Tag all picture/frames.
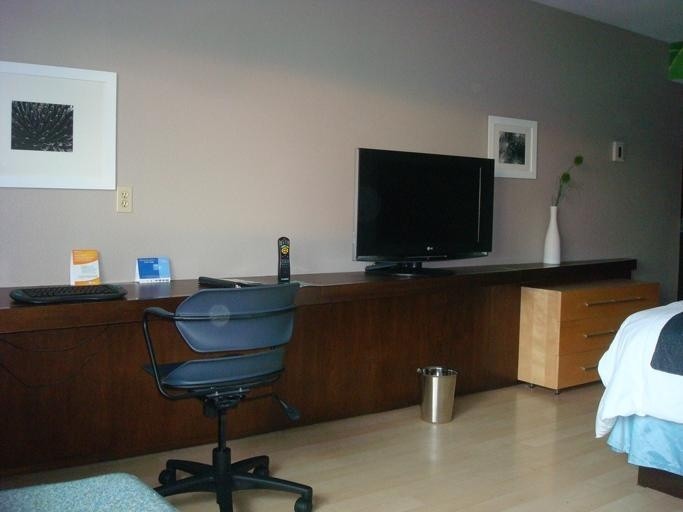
[0,60,119,193]
[486,114,538,181]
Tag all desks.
[0,257,638,480]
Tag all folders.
[200,276,260,289]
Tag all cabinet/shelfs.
[516,279,660,396]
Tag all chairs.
[139,282,312,511]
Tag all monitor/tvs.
[351,146,496,277]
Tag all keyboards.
[9,283,129,305]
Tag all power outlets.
[115,184,133,214]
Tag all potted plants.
[543,155,584,264]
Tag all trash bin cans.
[417,366,459,424]
